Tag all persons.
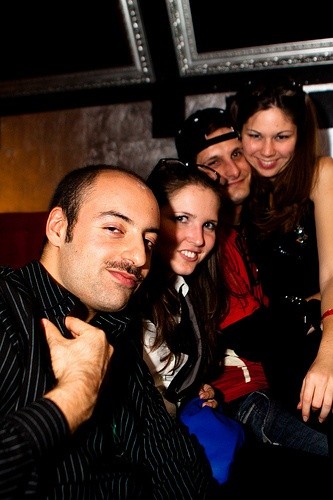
[142,156,245,481]
[168,75,333,500]
[0,162,234,500]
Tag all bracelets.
[320,308,333,318]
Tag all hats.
[176,108,241,163]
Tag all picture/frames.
[0,0,155,97]
[166,0,333,76]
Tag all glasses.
[148,159,222,188]
